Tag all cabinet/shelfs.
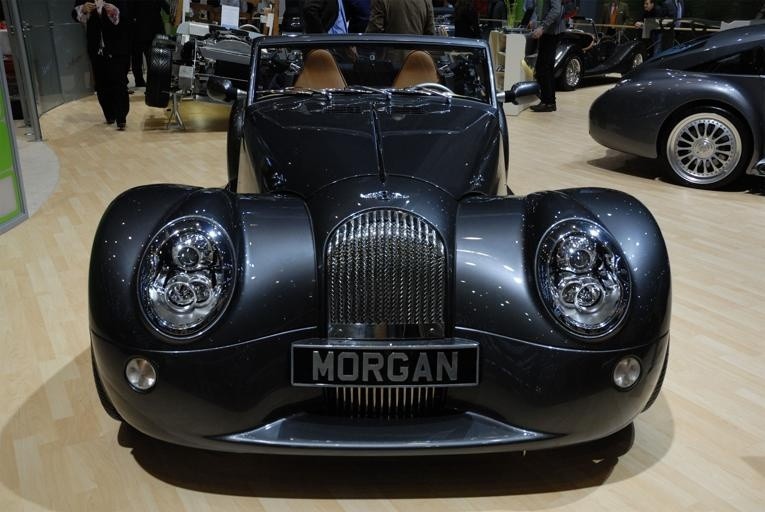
[488,29,540,116]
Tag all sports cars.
[589,24,765,188]
[554,18,645,90]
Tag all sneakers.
[106,118,127,128]
[530,99,557,113]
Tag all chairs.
[391,50,440,93]
[291,50,349,90]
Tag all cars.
[89,33,670,456]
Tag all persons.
[659,0,689,48]
[127,0,170,87]
[597,0,631,40]
[526,0,567,113]
[300,0,358,62]
[71,0,131,131]
[633,0,665,61]
[451,1,481,96]
[489,0,508,29]
[366,1,436,71]
